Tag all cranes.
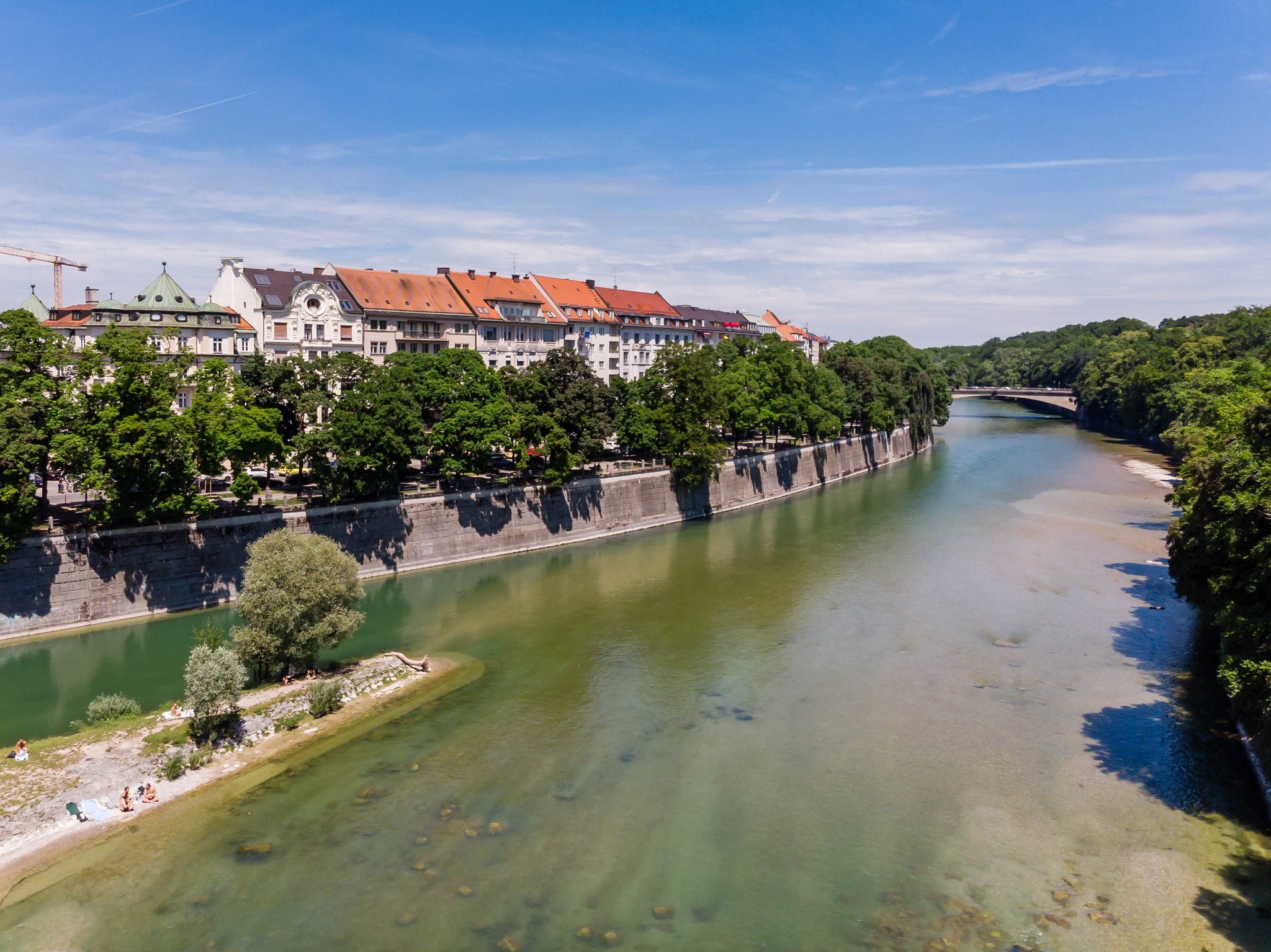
[0,245,89,309]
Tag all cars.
[192,479,232,494]
[188,476,198,483]
[605,441,620,451]
[241,466,276,478]
[970,386,978,389]
[420,446,550,474]
[28,473,43,487]
[46,469,62,481]
[326,461,339,470]
[285,473,320,486]
[1001,387,1011,389]
[387,464,420,475]
[198,474,232,481]
[601,446,630,457]
[278,465,309,477]
[251,476,284,487]
[714,423,763,441]
[1045,387,1052,391]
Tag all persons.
[59,478,74,493]
[15,739,28,757]
[283,674,295,685]
[142,783,159,804]
[591,463,602,473]
[120,786,135,812]
[171,703,181,717]
[751,439,756,453]
[306,668,316,680]
